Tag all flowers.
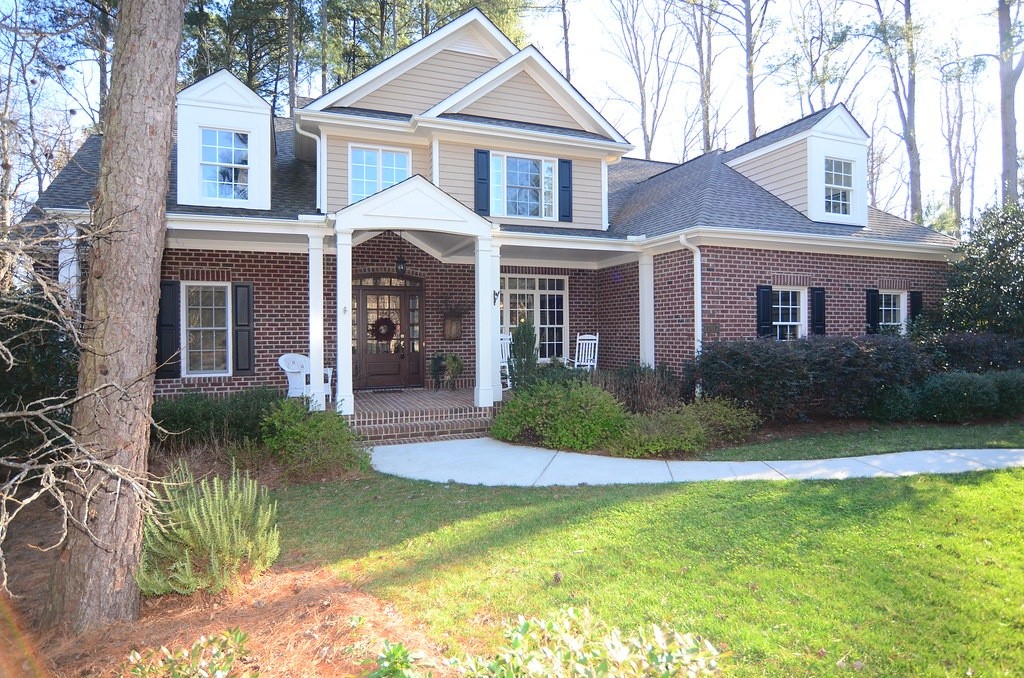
[369,317,396,342]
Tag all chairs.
[563,333,599,370]
[497,332,512,391]
[278,352,333,406]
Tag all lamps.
[395,228,408,274]
